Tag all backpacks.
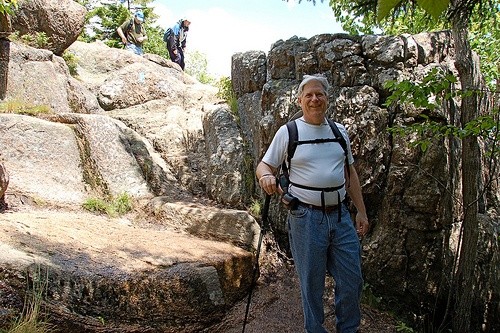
[163,27,173,42]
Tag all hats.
[134,11,145,22]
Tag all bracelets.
[261,174,272,177]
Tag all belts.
[300,200,349,211]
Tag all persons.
[255,75,369,333]
[117,12,148,56]
[167,16,192,71]
